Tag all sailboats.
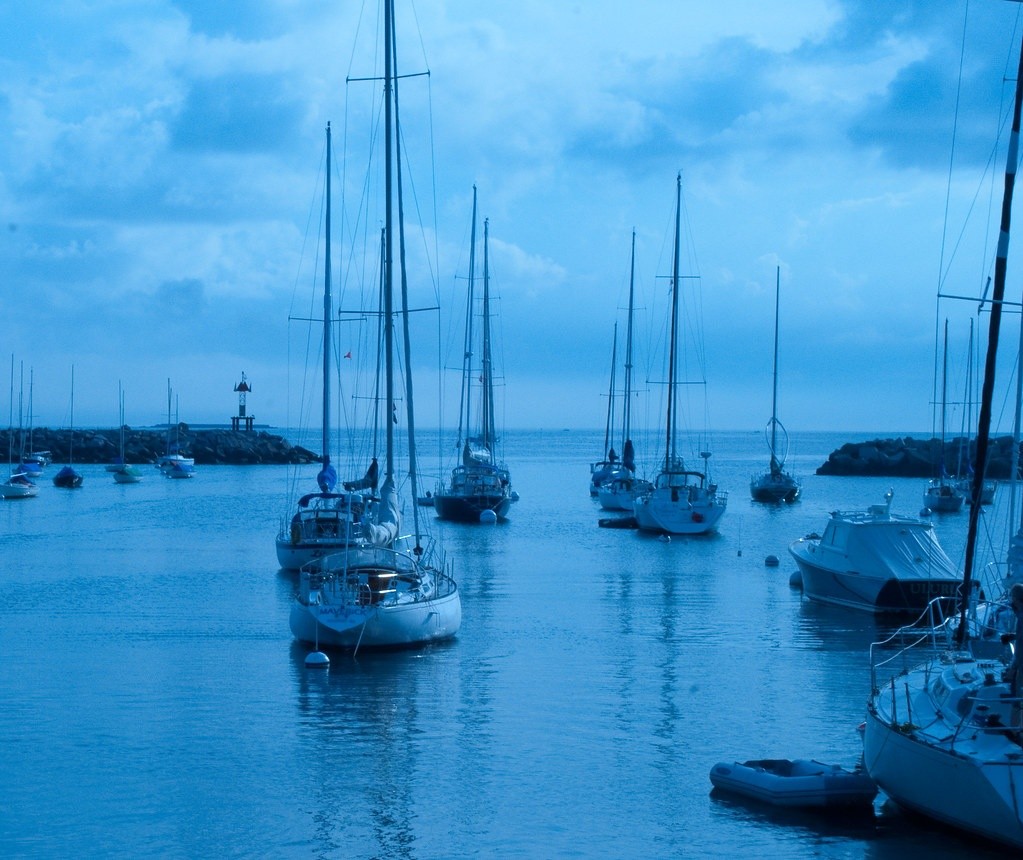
[750,265,803,503]
[160,394,197,479]
[154,377,195,469]
[113,390,145,484]
[290,0,460,649]
[924,318,966,512]
[435,218,513,523]
[856,0,1023,860]
[599,228,655,511]
[52,365,84,488]
[0,354,41,498]
[590,321,636,497]
[276,121,404,574]
[11,365,52,476]
[632,170,728,536]
[416,183,477,507]
[105,380,132,471]
[953,318,995,504]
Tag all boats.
[710,759,878,807]
[789,486,986,616]
